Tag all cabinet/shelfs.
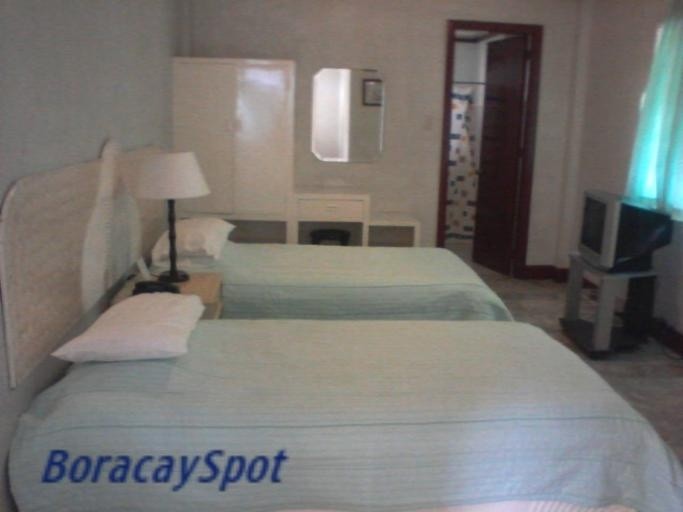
[172,56,295,244]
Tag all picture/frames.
[363,79,382,106]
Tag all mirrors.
[311,68,385,161]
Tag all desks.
[293,185,420,247]
[560,254,659,360]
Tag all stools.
[310,230,349,246]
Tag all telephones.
[132,281,180,297]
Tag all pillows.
[152,216,237,262]
[51,292,205,364]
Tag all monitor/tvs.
[579,190,671,272]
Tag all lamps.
[132,153,210,282]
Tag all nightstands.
[111,274,223,320]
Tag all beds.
[7,319,683,512]
[147,243,514,320]
